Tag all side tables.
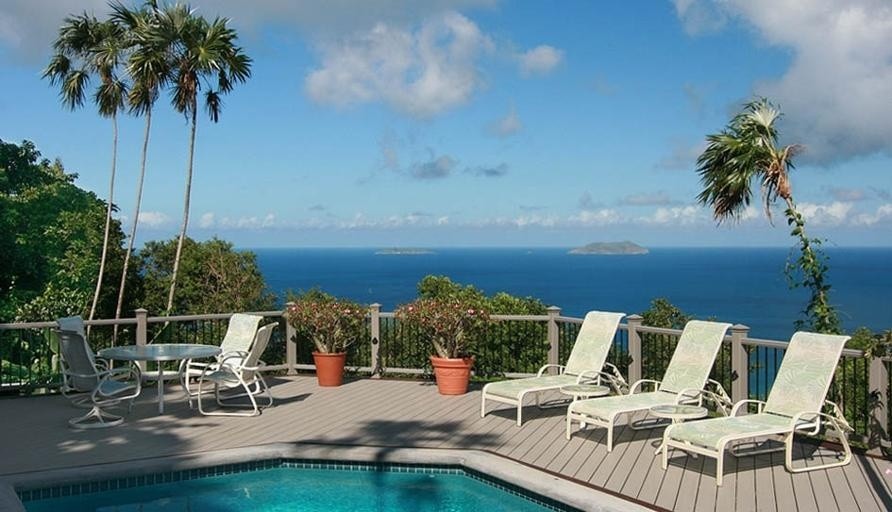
[560,384,610,429]
[648,404,708,459]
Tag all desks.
[97,342,222,414]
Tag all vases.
[430,355,476,396]
[312,352,347,387]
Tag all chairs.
[567,319,734,452]
[185,313,279,418]
[481,310,627,427]
[51,315,138,429]
[663,331,854,485]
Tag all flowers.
[393,295,484,358]
[282,297,370,354]
